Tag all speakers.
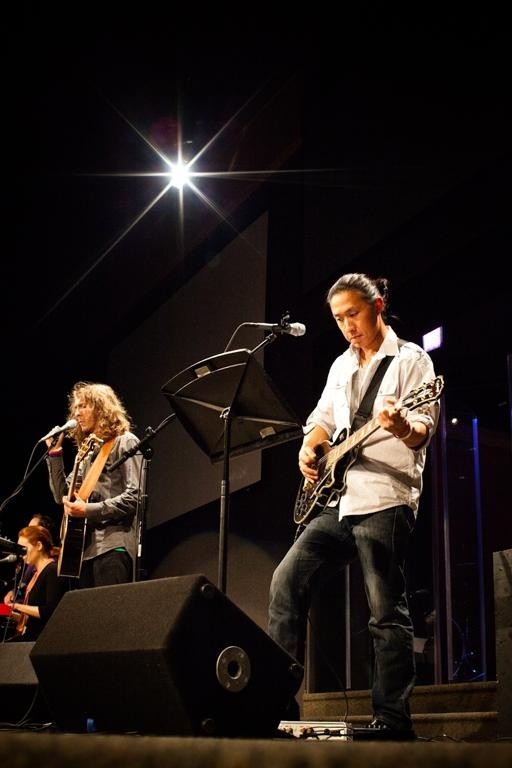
[27,572,307,734]
[0,639,52,729]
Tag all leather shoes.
[369,719,416,742]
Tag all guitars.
[56,431,105,579]
[0,602,28,635]
[293,375,446,525]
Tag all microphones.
[245,317,310,339]
[0,546,18,571]
[147,409,180,437]
[39,419,79,442]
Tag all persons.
[1,525,78,648]
[28,513,61,555]
[40,378,153,588]
[258,269,444,744]
